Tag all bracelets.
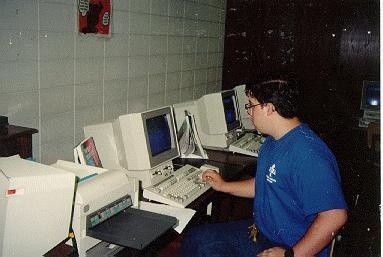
[284,248,294,257]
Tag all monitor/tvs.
[172,90,241,135]
[84,105,180,171]
[360,80,380,111]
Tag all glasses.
[245,104,261,117]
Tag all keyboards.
[229,133,266,157]
[143,165,213,208]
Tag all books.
[73,136,103,168]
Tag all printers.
[48,162,177,257]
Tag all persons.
[180,73,348,257]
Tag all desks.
[114,150,257,257]
